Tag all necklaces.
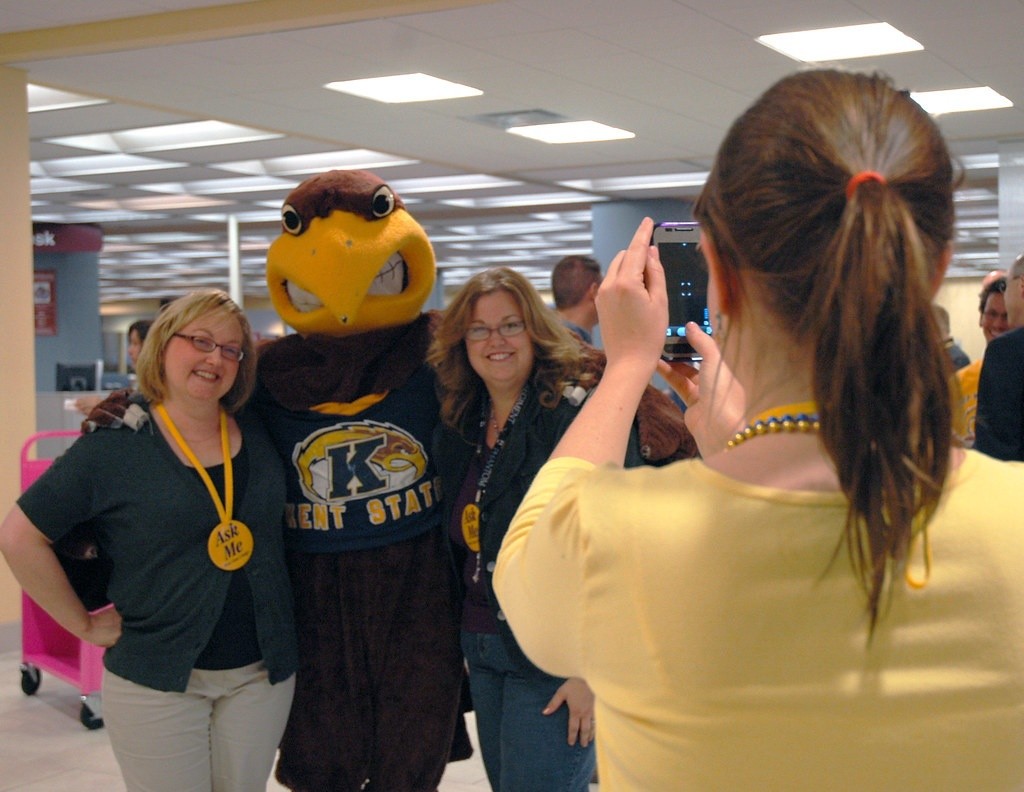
[724,402,821,451]
[184,427,218,443]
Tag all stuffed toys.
[81,169,697,792]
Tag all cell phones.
[650,222,713,363]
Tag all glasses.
[464,321,525,341]
[172,333,244,362]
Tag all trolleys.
[19,429,116,729]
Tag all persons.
[491,70,1024,792]
[0,287,300,792]
[550,256,603,345]
[424,268,646,792]
[127,319,151,365]
[930,256,1024,462]
